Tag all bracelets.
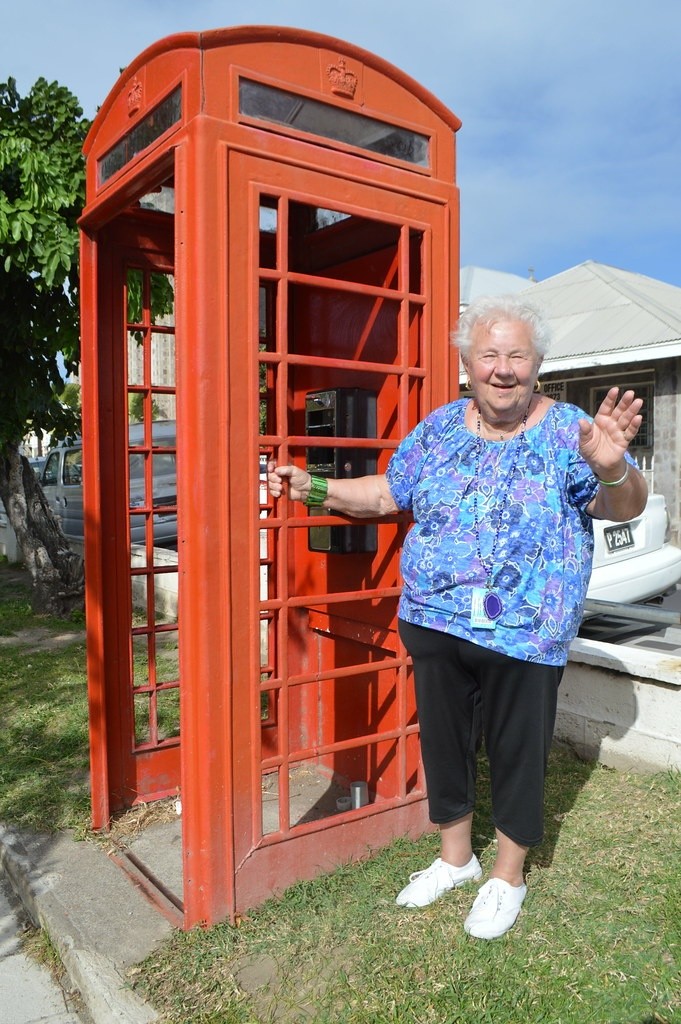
[303,475,328,508]
[593,463,630,487]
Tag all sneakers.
[396,853,482,908]
[464,878,528,940]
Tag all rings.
[624,433,631,441]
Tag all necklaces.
[483,417,520,440]
[475,402,530,617]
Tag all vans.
[36,419,177,545]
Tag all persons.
[258,293,648,941]
